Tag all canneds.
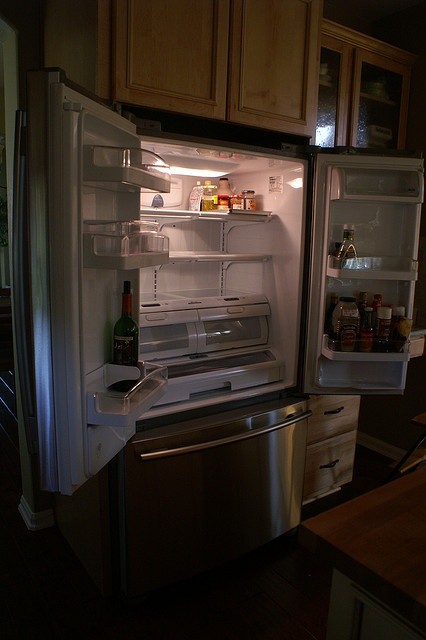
[217,194,229,210]
[360,326,374,351]
[242,190,255,211]
[229,194,242,210]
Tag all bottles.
[217,178,234,213]
[113,281,139,391]
[330,292,406,352]
[202,180,216,212]
[337,224,358,269]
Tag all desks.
[301,468,425,639]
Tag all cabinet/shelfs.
[312,19,412,147]
[44,0,321,138]
[304,393,360,505]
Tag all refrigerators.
[14,66,424,594]
